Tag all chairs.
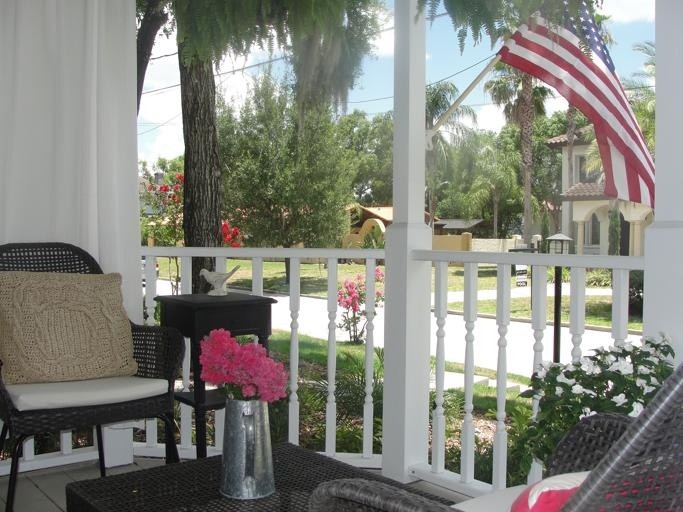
[0,243,185,512]
[309,362,682,512]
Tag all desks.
[66,442,460,512]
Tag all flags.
[493,3,654,209]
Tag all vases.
[218,398,276,500]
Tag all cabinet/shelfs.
[157,283,274,465]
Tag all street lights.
[544,234,573,363]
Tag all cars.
[141,257,159,286]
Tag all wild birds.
[199,265,240,289]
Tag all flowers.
[197,325,287,405]
